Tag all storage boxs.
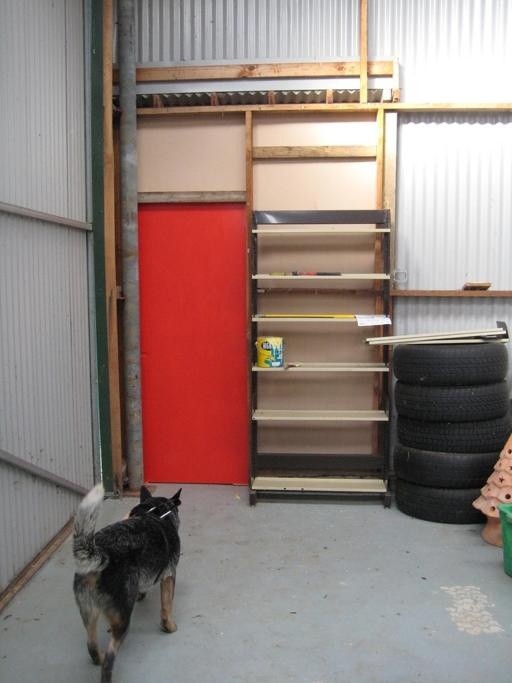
[496,502,512,578]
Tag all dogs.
[70,482,182,683]
